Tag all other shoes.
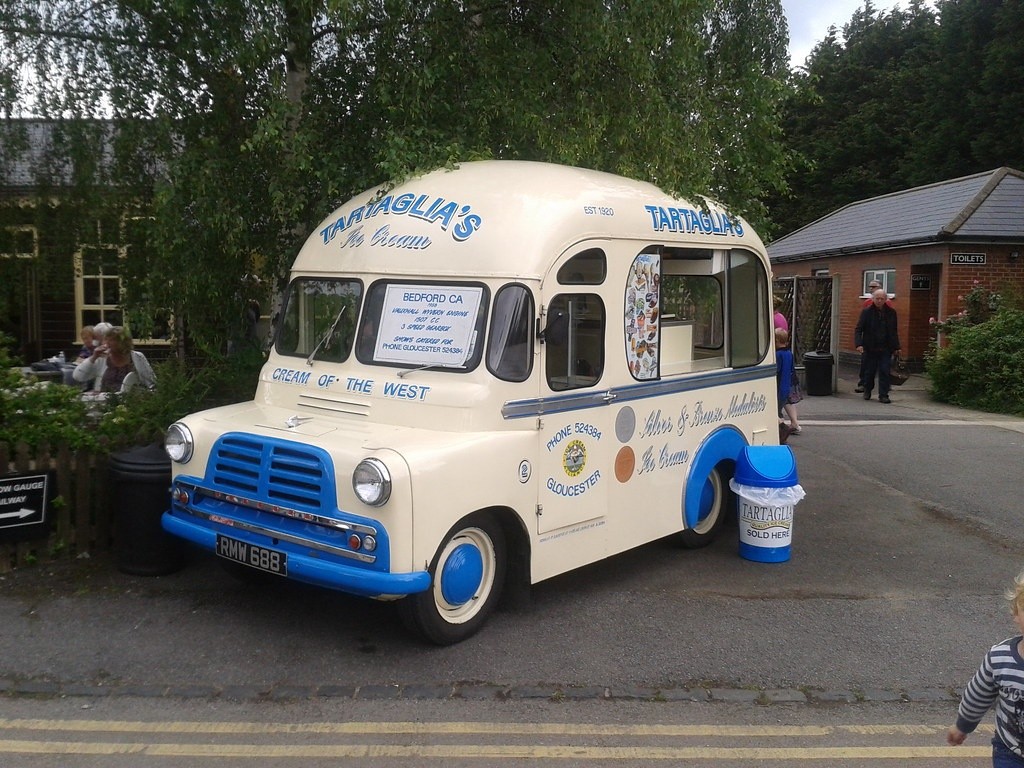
[790,426,802,435]
[855,385,866,393]
[863,388,872,400]
[878,393,891,404]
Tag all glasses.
[869,286,880,288]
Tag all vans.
[160,159,781,648]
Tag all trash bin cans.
[109,442,185,576]
[802,350,834,396]
[734,445,798,563]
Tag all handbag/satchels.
[887,353,910,386]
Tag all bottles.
[59,351,65,363]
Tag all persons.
[947,574,1024,768]
[854,289,900,403]
[774,310,803,434]
[72,323,158,392]
[774,328,793,422]
[855,279,894,393]
